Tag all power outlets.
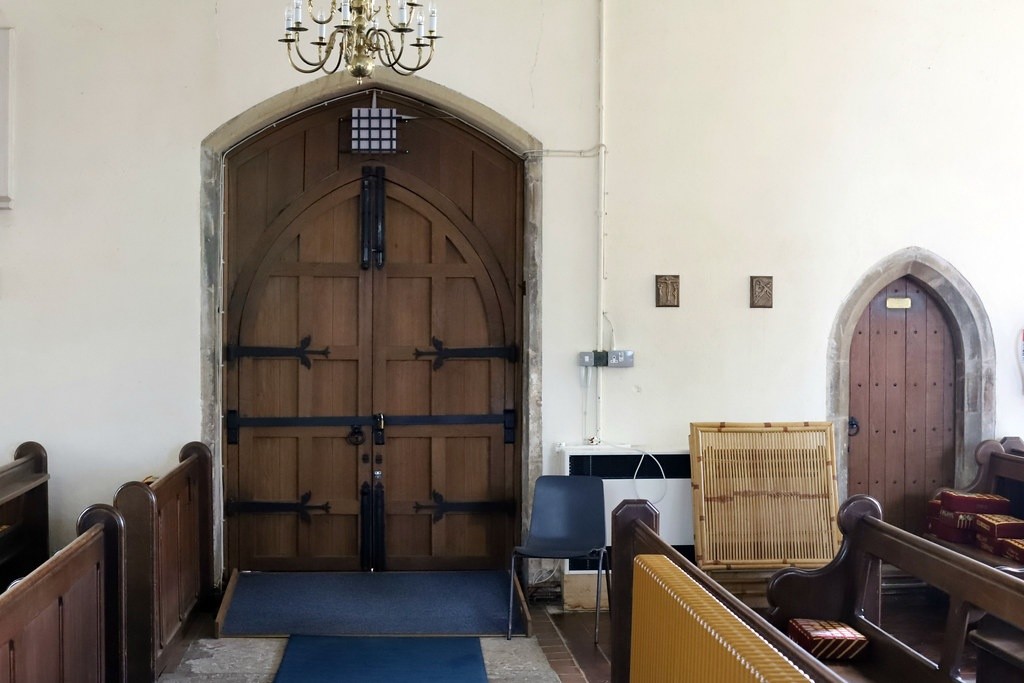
[607,351,636,366]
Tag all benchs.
[767,437,1024,683]
[0,441,212,683]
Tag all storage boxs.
[784,617,873,661]
[929,491,1024,563]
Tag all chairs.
[507,474,613,647]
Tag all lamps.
[280,1,442,88]
